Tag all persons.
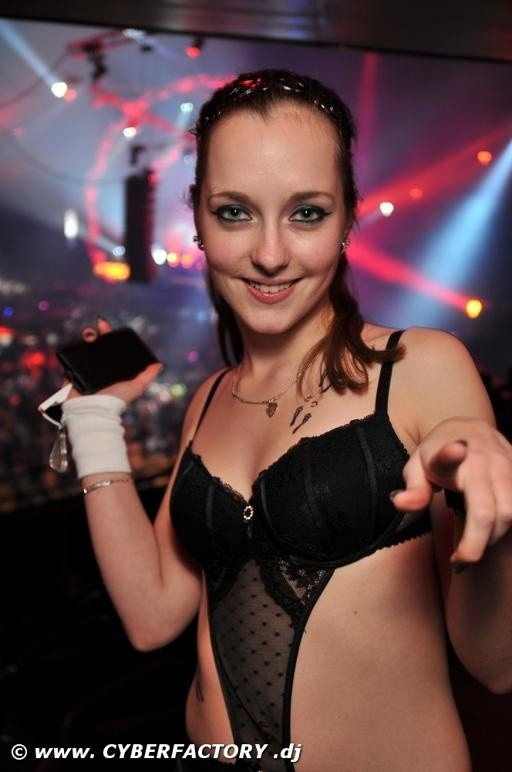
[48,67,512,772]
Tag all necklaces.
[231,358,309,417]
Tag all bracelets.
[80,478,135,494]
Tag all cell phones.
[54,323,155,391]
